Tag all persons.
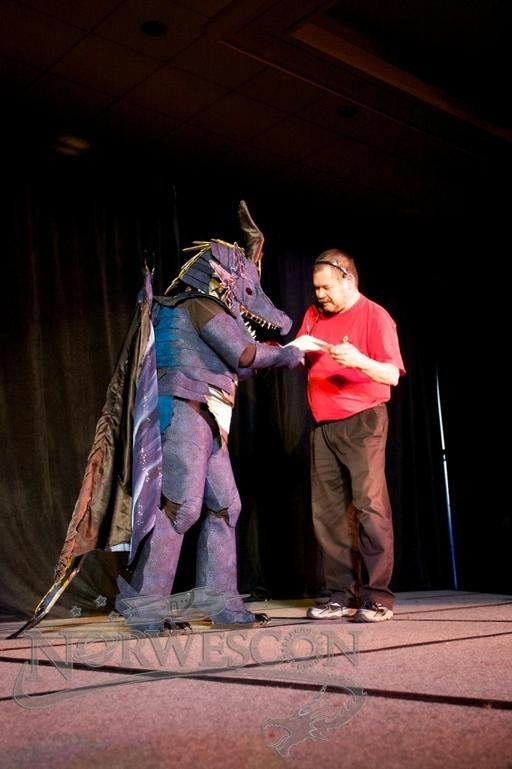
[7,199,306,637]
[289,248,401,623]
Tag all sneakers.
[307,600,358,619]
[355,600,394,623]
